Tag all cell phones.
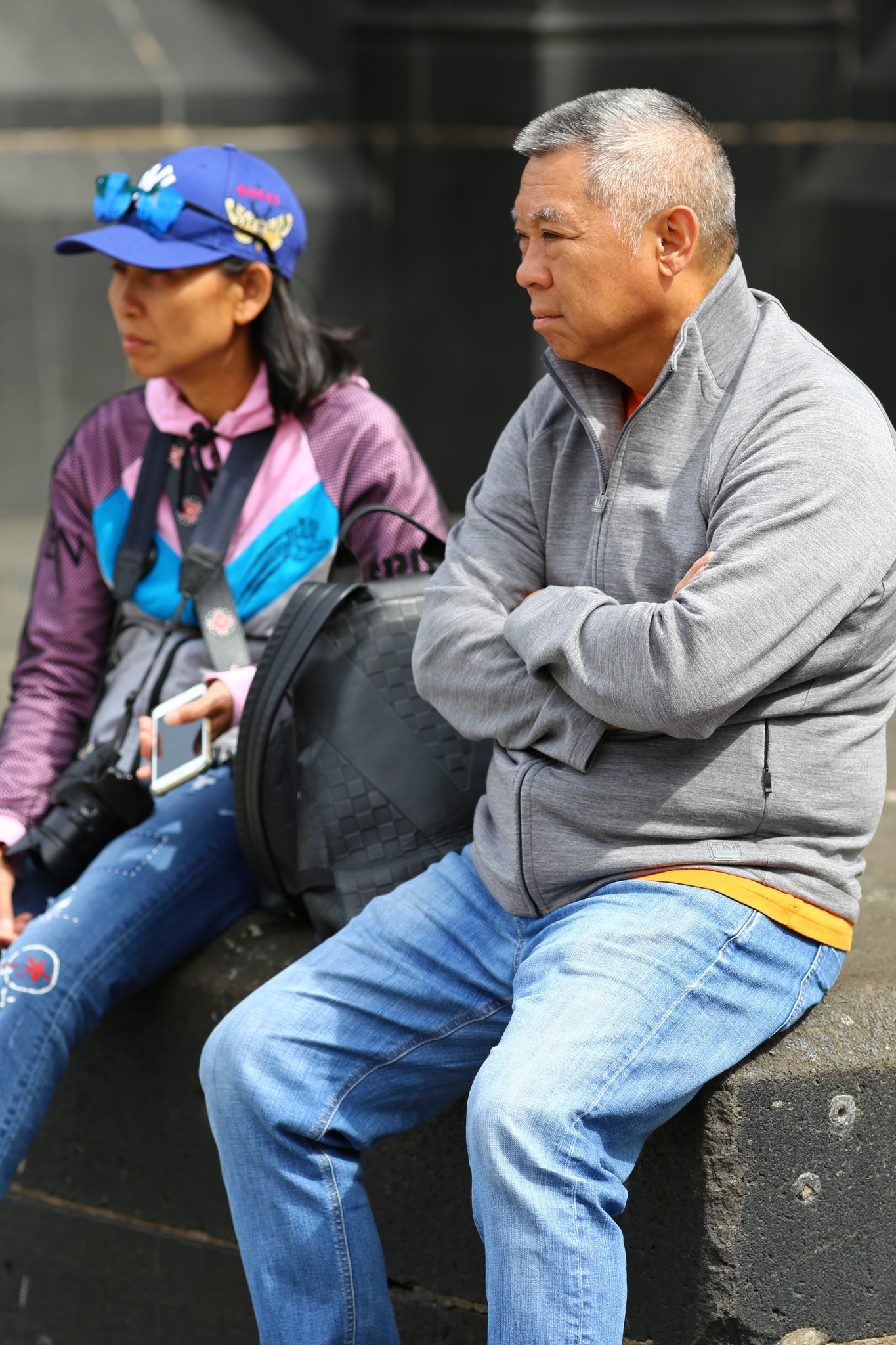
[150,682,214,797]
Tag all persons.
[197,86,896,1345]
[0,147,448,1179]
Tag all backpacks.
[233,503,496,944]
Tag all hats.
[54,143,308,282]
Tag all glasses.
[94,171,276,273]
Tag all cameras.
[2,769,157,898]
